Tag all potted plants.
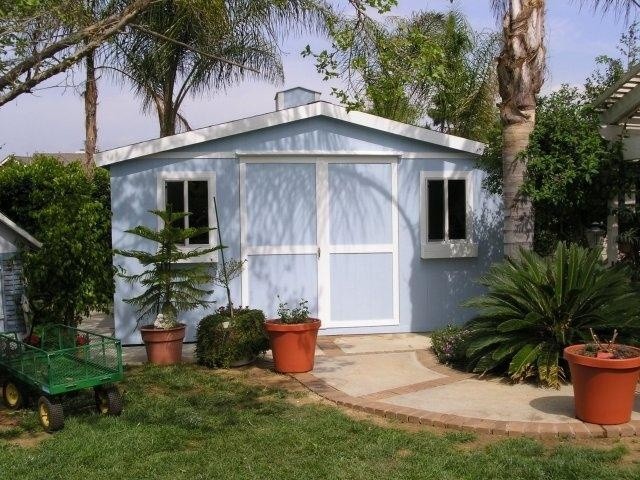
[564,326,640,424]
[113,202,322,378]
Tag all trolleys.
[1,323,125,434]
